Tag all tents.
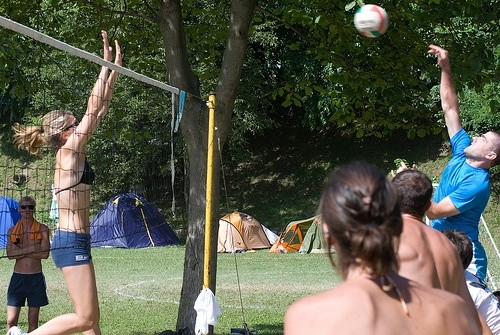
[89,192,180,249]
[0,195,22,248]
[217,211,284,253]
[268,213,336,254]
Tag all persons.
[442,229,500,335]
[391,169,483,335]
[7,197,51,333]
[5,32,123,335]
[392,44,500,283]
[284,160,478,334]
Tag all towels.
[194,287,221,335]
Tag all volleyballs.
[353,4,390,39]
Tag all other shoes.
[7,326,26,335]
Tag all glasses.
[63,121,79,131]
[21,205,35,209]
